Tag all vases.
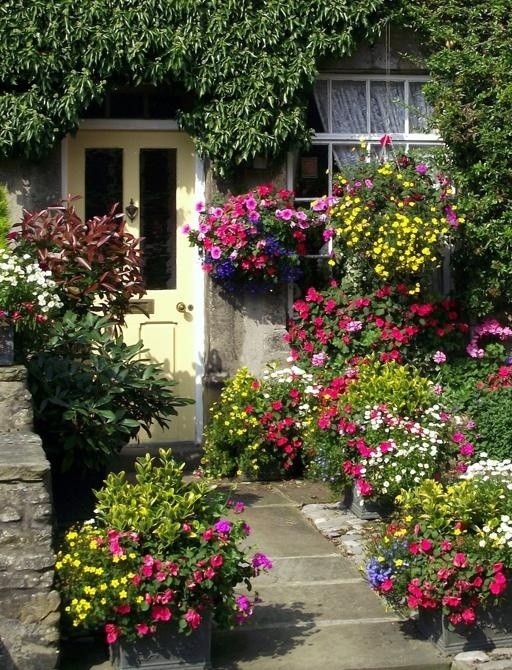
[416,602,512,657]
[108,614,213,670]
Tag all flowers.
[52,128,512,644]
